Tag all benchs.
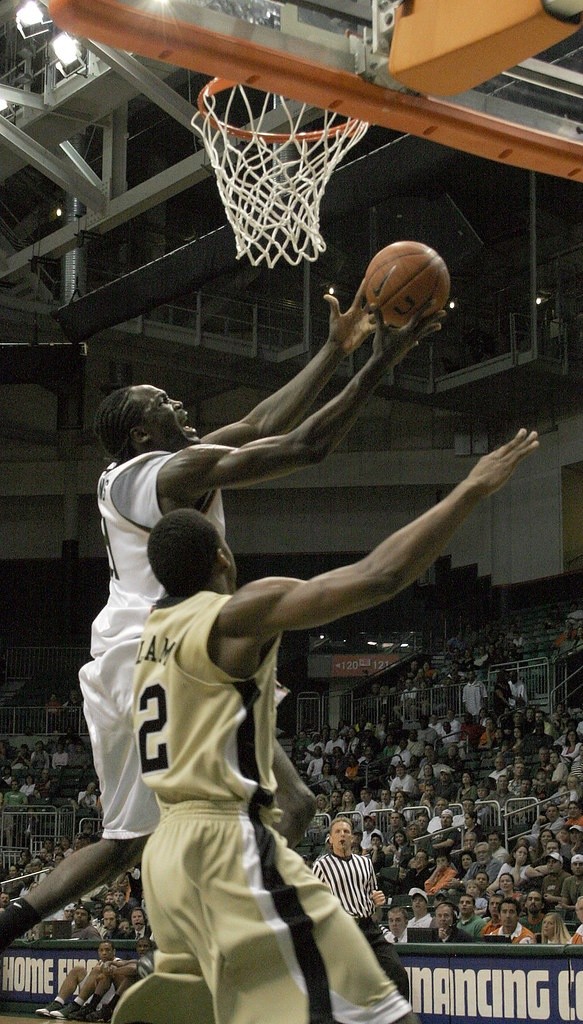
[0,595,583,933]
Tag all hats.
[434,888,449,899]
[440,767,451,776]
[545,852,563,864]
[537,767,547,773]
[77,904,92,921]
[409,888,428,903]
[64,903,76,911]
[364,816,376,825]
[571,854,583,864]
[568,825,583,832]
[442,809,453,818]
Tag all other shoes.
[86,1003,114,1023]
[69,1004,96,1020]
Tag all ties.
[394,937,398,943]
[135,933,140,940]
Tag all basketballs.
[363,240,451,327]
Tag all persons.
[132,429,537,1024]
[0,611,582,1021]
[2,278,442,942]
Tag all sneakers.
[50,1002,80,1019]
[35,1000,64,1016]
